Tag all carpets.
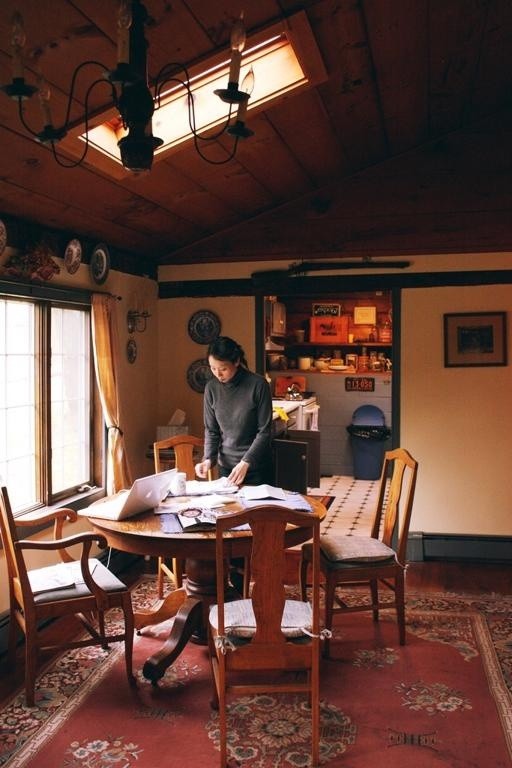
[306,494,337,513]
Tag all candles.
[133,301,148,314]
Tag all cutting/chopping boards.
[274,376,306,397]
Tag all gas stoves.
[270,395,316,406]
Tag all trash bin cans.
[346,405,386,480]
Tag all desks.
[83,491,329,693]
[144,442,199,472]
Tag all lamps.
[56,10,333,185]
[0,1,258,176]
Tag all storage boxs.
[310,315,349,344]
[311,303,340,317]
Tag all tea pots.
[285,383,304,401]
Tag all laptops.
[77,468,178,521]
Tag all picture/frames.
[353,306,376,324]
[442,308,508,370]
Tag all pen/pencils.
[162,490,172,503]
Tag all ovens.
[299,402,320,431]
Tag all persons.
[194,336,273,599]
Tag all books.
[175,507,217,532]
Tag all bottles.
[383,319,391,329]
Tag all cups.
[373,361,381,372]
[173,472,187,496]
[298,358,311,370]
[368,333,375,341]
[294,329,305,343]
[348,334,354,343]
[280,356,287,370]
[314,359,325,370]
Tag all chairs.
[0,484,139,707]
[207,503,322,768]
[297,447,420,660]
[151,433,216,600]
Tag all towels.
[310,404,319,431]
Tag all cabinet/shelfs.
[264,298,287,337]
[270,406,321,496]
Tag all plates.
[87,243,110,286]
[188,309,222,345]
[63,238,82,276]
[0,220,7,256]
[186,359,216,394]
[329,365,349,370]
[125,340,139,365]
[126,310,136,334]
